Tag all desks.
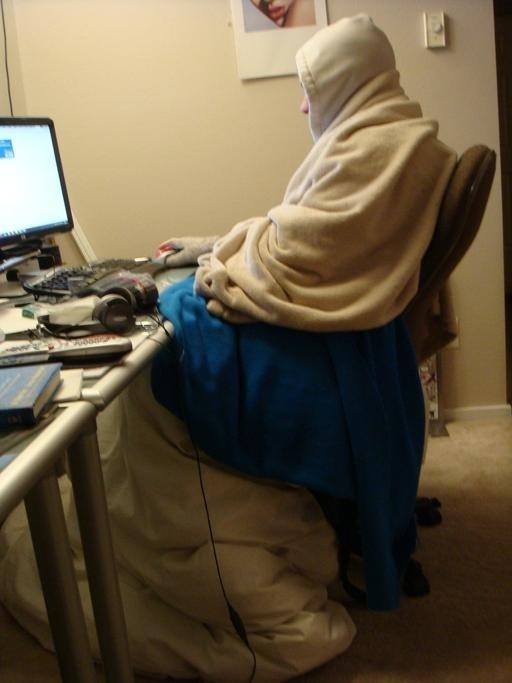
[1,262,203,683]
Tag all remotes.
[48,336,132,360]
[0,334,49,367]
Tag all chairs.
[154,138,498,594]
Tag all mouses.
[150,246,179,267]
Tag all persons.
[0,10,457,680]
[244,2,315,30]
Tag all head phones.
[37,286,138,338]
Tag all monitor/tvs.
[0,117,74,258]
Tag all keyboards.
[23,257,165,297]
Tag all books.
[0,356,85,457]
